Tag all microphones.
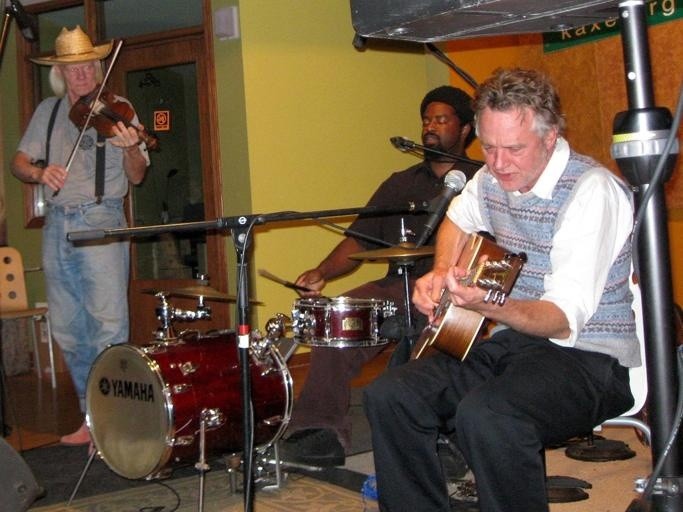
[414,169,467,250]
[390,135,416,152]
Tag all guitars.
[408,234,526,361]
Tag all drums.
[292,296,392,347]
[85,326,296,480]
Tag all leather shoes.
[60,421,91,444]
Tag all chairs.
[564,262,655,462]
[0,245,57,391]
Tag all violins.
[68,81,157,150]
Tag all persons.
[11,23,150,461]
[262,82,489,467]
[360,63,653,511]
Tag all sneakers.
[240,428,346,473]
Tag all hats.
[29,24,114,65]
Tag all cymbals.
[141,280,267,309]
[344,235,438,267]
